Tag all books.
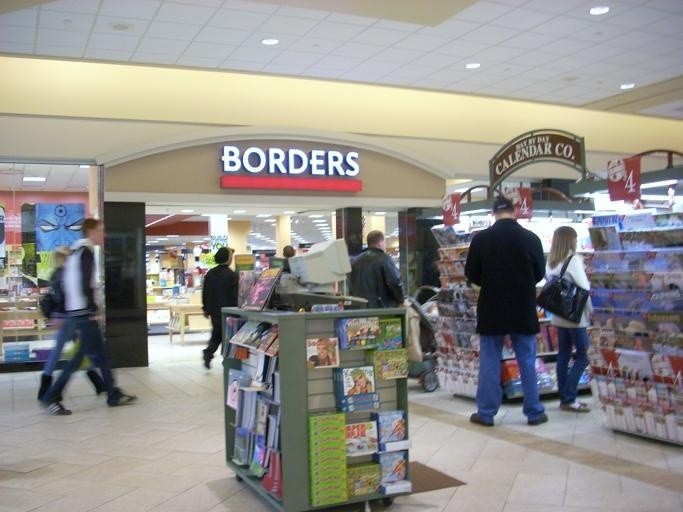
[220,314,281,501]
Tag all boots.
[86,370,107,395]
[37,373,65,402]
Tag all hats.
[214,248,235,265]
[493,197,513,212]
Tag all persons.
[347,368,372,396]
[40,218,137,416]
[280,246,297,275]
[199,246,238,370]
[347,230,405,308]
[462,198,548,427]
[307,338,337,367]
[36,245,122,402]
[542,225,594,414]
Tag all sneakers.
[562,400,591,413]
[559,400,587,409]
[106,388,139,406]
[39,398,71,414]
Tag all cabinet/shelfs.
[431,211,589,406]
[584,212,683,448]
[221,305,411,512]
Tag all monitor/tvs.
[288,238,352,293]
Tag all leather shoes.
[471,411,494,426]
[527,414,549,425]
[203,350,211,368]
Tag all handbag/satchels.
[537,275,590,323]
[41,292,53,316]
